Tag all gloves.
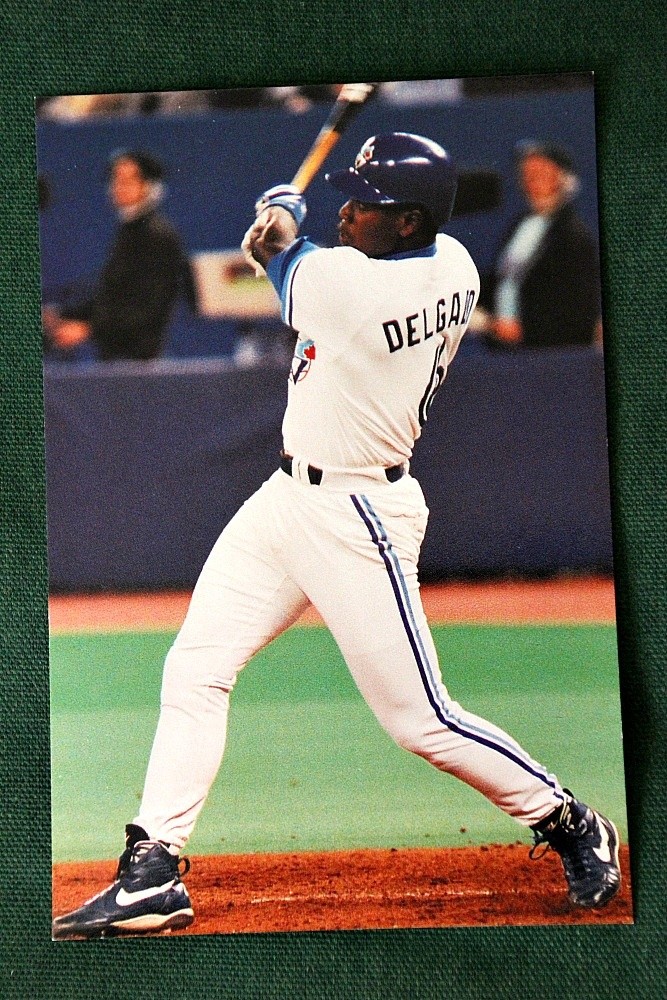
[253,183,307,226]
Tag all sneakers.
[529,788,621,908]
[51,824,195,938]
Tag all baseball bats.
[251,81,379,264]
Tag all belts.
[280,449,405,485]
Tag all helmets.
[324,133,457,225]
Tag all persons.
[467,136,600,350]
[50,127,623,941]
[42,148,185,363]
